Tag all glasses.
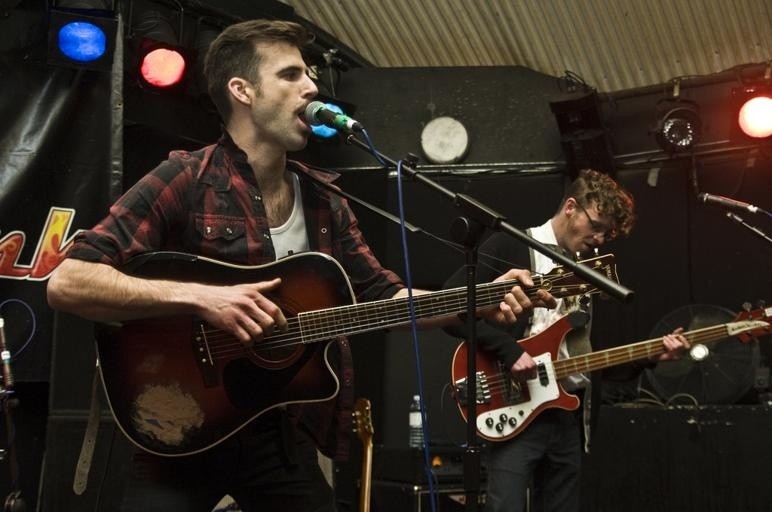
[575,197,615,243]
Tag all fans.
[644,302,761,411]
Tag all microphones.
[303,100,365,136]
[693,188,762,216]
[722,206,765,238]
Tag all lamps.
[727,65,772,141]
[646,77,701,155]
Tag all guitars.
[94,247,620,457]
[352,396,375,510]
[451,301,772,441]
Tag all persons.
[45,17,560,510]
[437,165,693,512]
